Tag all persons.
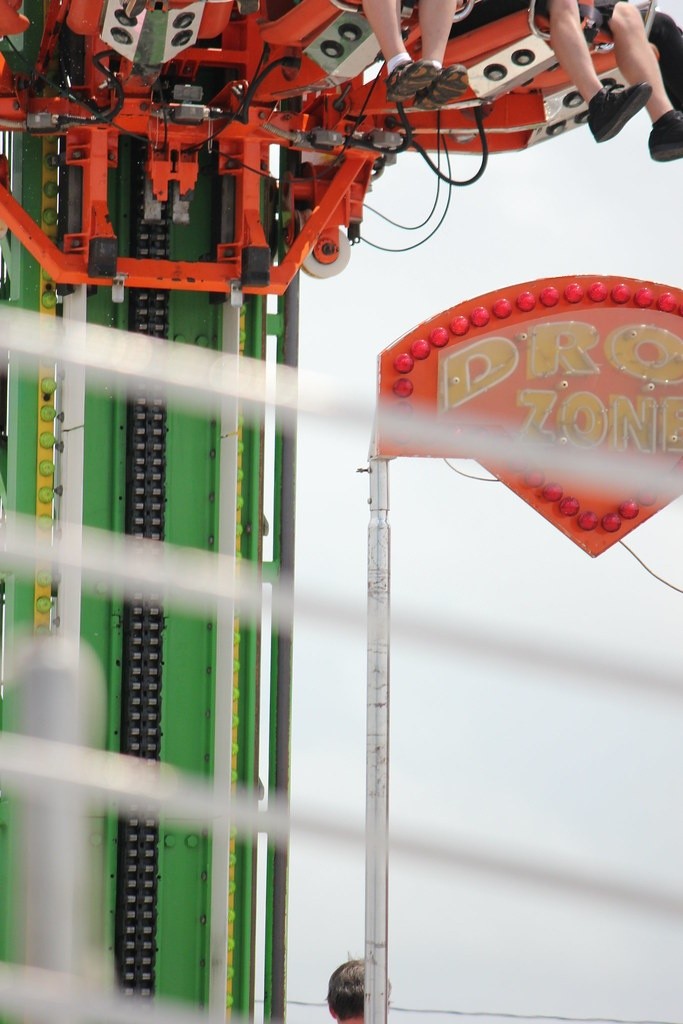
[325,959,392,1024]
[534,0,683,162]
[362,0,468,111]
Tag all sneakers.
[413,65,469,110]
[648,111,683,162]
[586,82,652,143]
[386,58,442,103]
